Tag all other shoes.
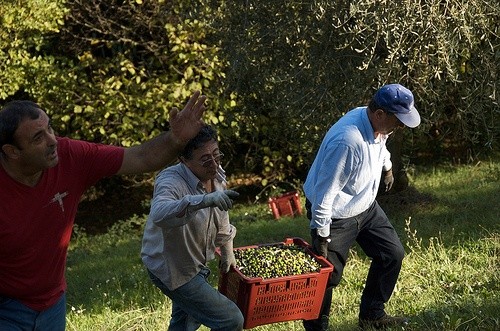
[359,314,406,330]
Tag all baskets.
[215,238,334,329]
[268,190,303,220]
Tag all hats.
[374,84,421,128]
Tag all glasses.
[201,150,224,168]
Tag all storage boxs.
[215,237,335,328]
[268,191,303,220]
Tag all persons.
[141,124,244,331]
[302,84,421,331]
[0,90,207,331]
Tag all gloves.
[218,241,236,273]
[312,237,327,259]
[382,168,394,193]
[205,190,239,211]
